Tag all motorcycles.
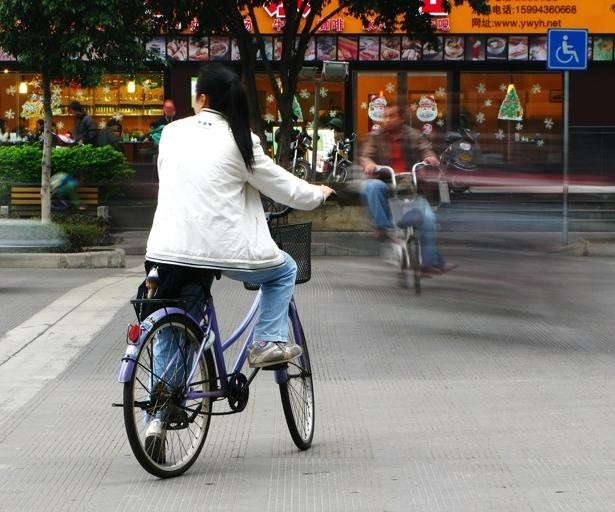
[439,123,481,193]
[323,120,357,185]
[49,128,85,149]
[275,115,313,181]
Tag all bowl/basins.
[487,37,506,54]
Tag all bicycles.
[364,159,439,295]
[111,185,338,480]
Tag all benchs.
[8,182,99,219]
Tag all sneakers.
[243,341,303,370]
[145,418,168,465]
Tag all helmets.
[163,98,177,116]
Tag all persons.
[360,101,462,275]
[141,65,337,467]
[0,100,177,157]
[248,107,299,164]
